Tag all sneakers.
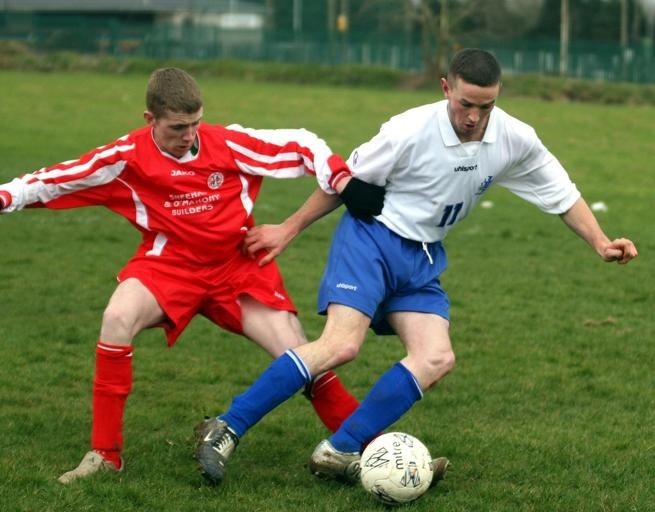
[192,414,240,484]
[57,451,126,486]
[431,456,452,489]
[308,438,364,483]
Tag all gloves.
[337,176,385,221]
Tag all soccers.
[359,432,434,505]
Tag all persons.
[0,65,452,493]
[191,43,641,488]
